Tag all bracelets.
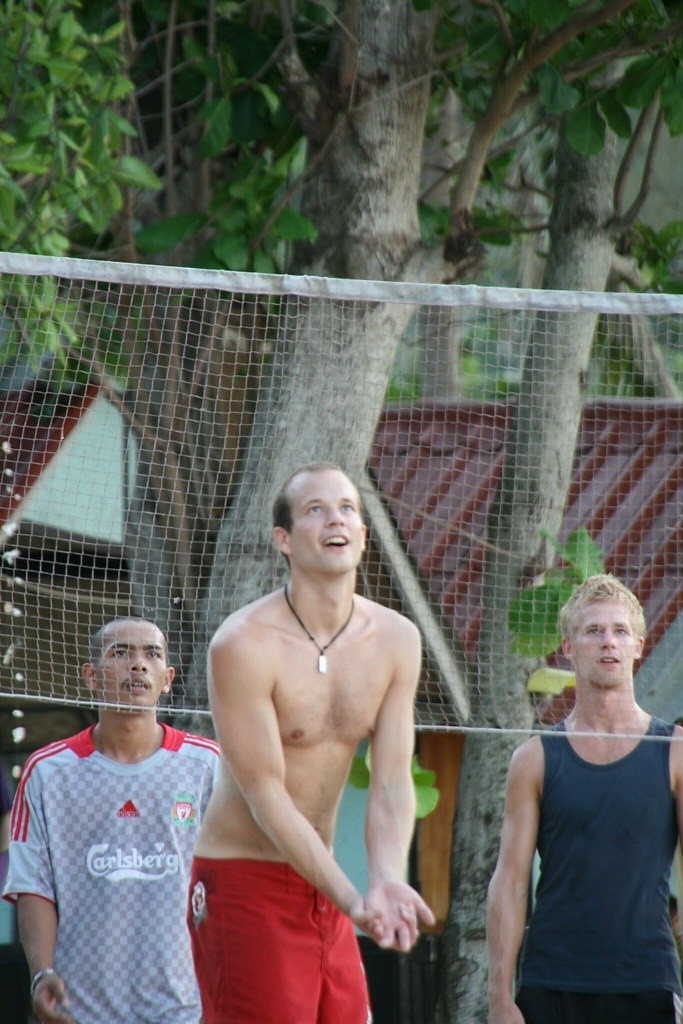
[27,967,59,997]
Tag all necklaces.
[277,583,355,675]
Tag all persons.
[182,458,437,1024]
[0,612,223,1024]
[485,572,682,1024]
